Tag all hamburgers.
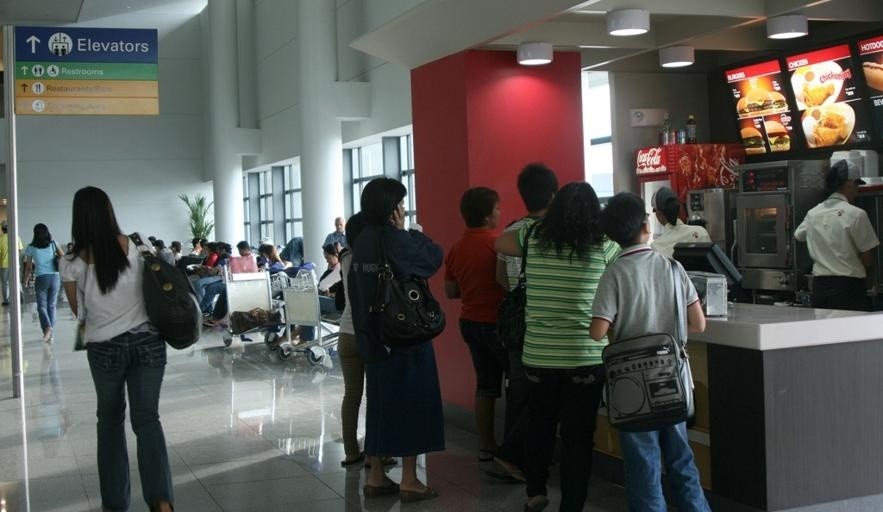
[739,126,766,156]
[763,120,790,152]
[735,87,789,118]
[862,61,883,92]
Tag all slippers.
[363,456,394,468]
[363,480,400,498]
[399,486,438,503]
[492,456,526,483]
[524,496,549,511]
[202,320,217,326]
[341,451,364,465]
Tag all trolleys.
[222,264,287,350]
[278,269,343,366]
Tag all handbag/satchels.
[496,221,540,351]
[330,252,352,311]
[258,309,281,325]
[52,240,61,272]
[601,258,694,433]
[230,308,264,334]
[364,244,446,362]
[129,233,203,350]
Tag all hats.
[832,159,866,185]
[651,186,678,213]
[0,220,8,228]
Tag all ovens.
[730,159,830,309]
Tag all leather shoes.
[2,302,9,305]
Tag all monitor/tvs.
[672,243,744,288]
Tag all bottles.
[662,127,668,145]
[657,128,663,145]
[663,111,673,130]
[677,127,686,144]
[686,113,697,143]
[668,127,677,143]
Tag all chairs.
[22,262,36,287]
[321,313,343,333]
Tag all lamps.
[606,10,651,36]
[516,41,554,65]
[659,45,694,68]
[766,15,808,40]
[2,197,7,205]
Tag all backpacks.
[51,242,67,274]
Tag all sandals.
[49,338,54,341]
[478,449,500,462]
[43,328,53,341]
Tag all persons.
[650,186,711,257]
[794,159,883,312]
[154,240,178,268]
[259,245,286,345]
[0,221,24,305]
[346,178,444,504]
[338,214,397,466]
[197,242,224,315]
[444,187,502,462]
[187,239,211,275]
[490,182,622,512]
[494,161,561,480]
[338,345,338,349]
[589,191,711,512]
[200,242,233,316]
[203,241,258,327]
[279,241,351,347]
[23,223,64,344]
[171,241,183,262]
[59,186,174,512]
[188,238,203,258]
[322,217,347,271]
